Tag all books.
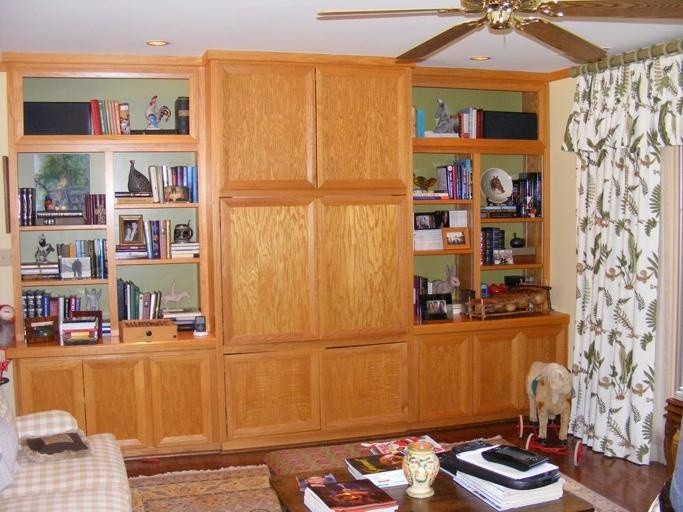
[452,471,566,511]
[21,262,59,281]
[411,103,553,327]
[22,287,111,346]
[175,96,189,135]
[143,220,172,259]
[117,278,201,331]
[371,433,444,463]
[116,245,148,259]
[170,244,199,258]
[295,451,411,512]
[18,188,36,226]
[84,194,106,225]
[91,100,131,135]
[57,238,108,280]
[115,165,198,204]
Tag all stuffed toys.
[0,304,16,386]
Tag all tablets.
[482,444,550,472]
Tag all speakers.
[23,101,91,135]
[484,110,538,140]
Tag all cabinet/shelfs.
[0,49,568,462]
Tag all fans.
[318,1,683,65]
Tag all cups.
[174,224,193,244]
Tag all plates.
[480,167,513,204]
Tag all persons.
[124,220,139,241]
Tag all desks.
[268,435,594,512]
[662,397,683,480]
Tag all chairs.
[0,386,133,512]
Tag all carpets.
[129,463,283,511]
